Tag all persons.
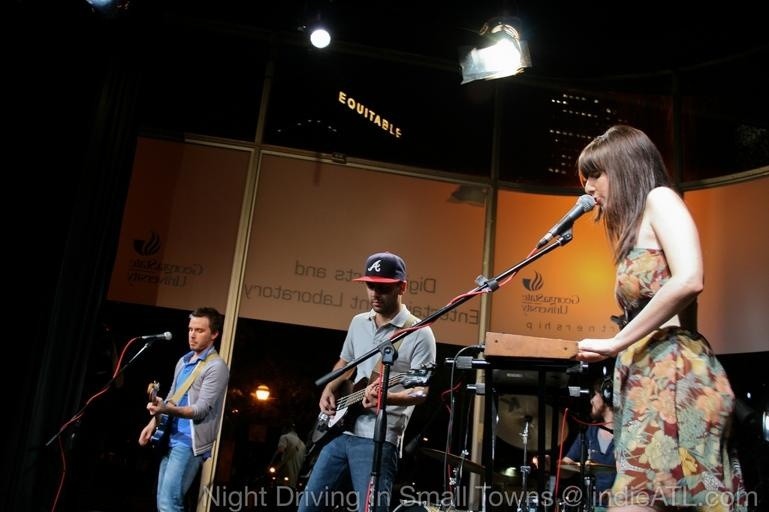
[137,305,229,512]
[555,373,619,504]
[298,251,439,510]
[263,421,307,486]
[570,121,740,512]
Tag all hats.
[353,250,407,284]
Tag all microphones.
[139,330,173,344]
[535,193,596,250]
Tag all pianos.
[482,332,578,362]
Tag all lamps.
[455,1,537,87]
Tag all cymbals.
[429,448,488,474]
[559,461,617,476]
[489,393,569,451]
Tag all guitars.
[148,382,173,460]
[312,369,431,446]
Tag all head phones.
[600,377,613,402]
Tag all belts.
[617,312,697,331]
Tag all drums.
[390,500,461,511]
